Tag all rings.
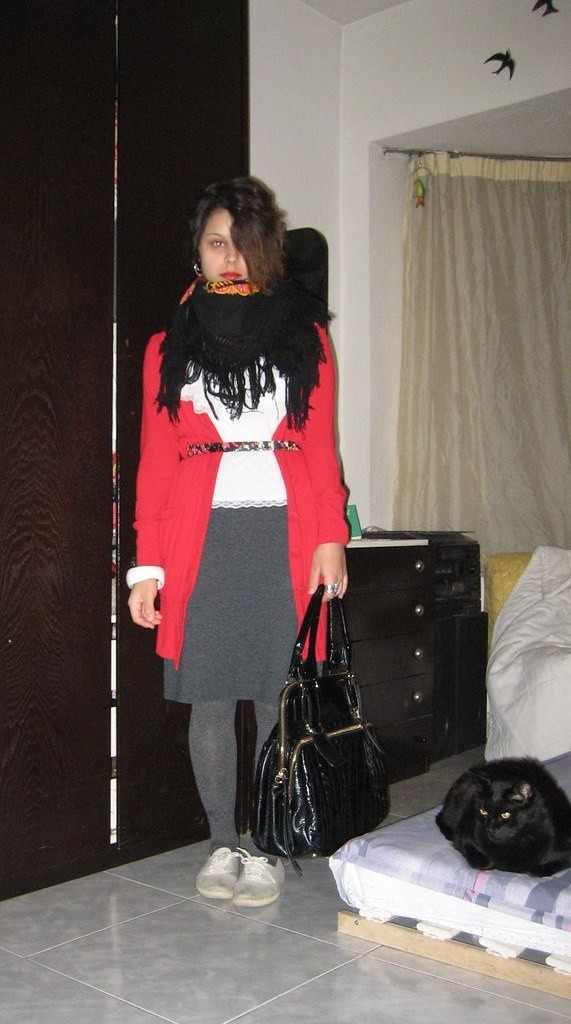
[325,583,340,593]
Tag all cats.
[435,757,571,878]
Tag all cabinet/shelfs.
[332,531,488,788]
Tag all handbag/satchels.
[251,583,390,860]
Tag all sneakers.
[195,846,240,899]
[233,847,285,906]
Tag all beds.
[329,751,571,1001]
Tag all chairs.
[485,549,533,648]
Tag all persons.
[126,179,348,906]
